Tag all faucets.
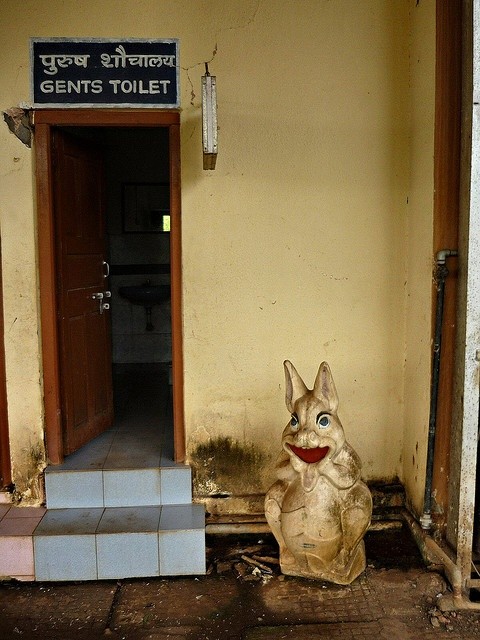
[146,279,150,286]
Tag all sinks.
[119,285,170,306]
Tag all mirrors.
[120,181,170,233]
